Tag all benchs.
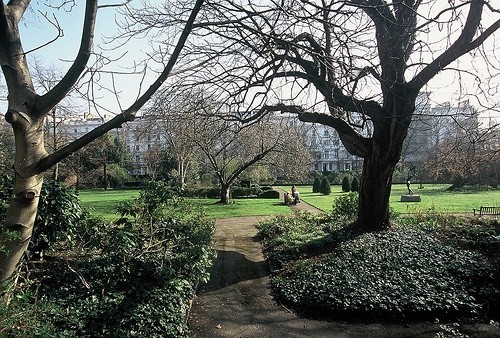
[285,195,294,206]
[472,206,500,218]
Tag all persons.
[292,185,298,198]
